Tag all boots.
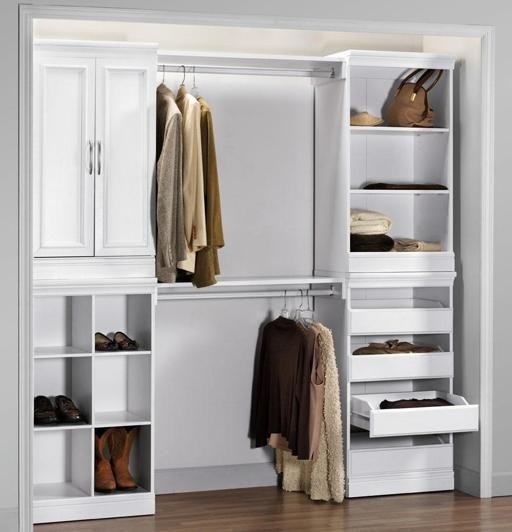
[95,427,138,493]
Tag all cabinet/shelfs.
[313,273,479,501]
[312,49,461,278]
[31,40,157,258]
[30,256,157,525]
[154,49,344,496]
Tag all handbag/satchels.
[386,69,443,127]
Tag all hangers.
[276,286,319,326]
[157,60,206,102]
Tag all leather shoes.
[95,332,118,351]
[114,331,138,350]
[55,394,82,423]
[34,396,58,425]
